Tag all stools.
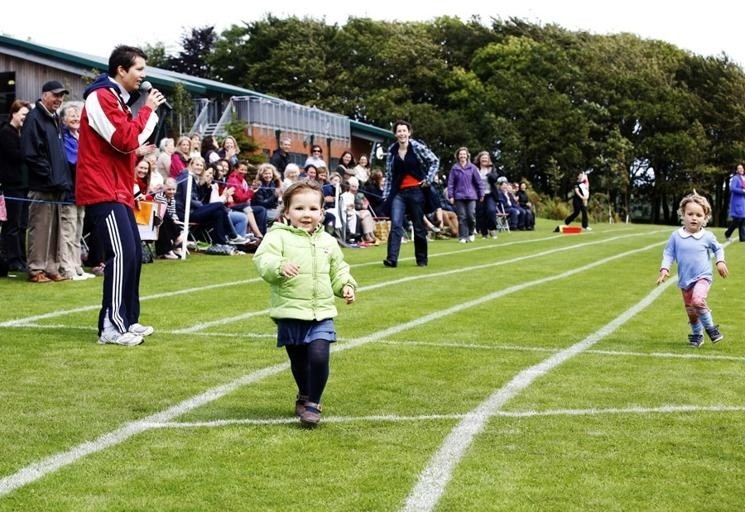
[496,212,511,235]
[185,223,217,249]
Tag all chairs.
[370,214,390,242]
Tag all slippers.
[164,250,182,259]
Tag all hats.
[42,81,70,95]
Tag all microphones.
[141,80,174,111]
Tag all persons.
[655,188,730,348]
[724,163,745,245]
[252,175,359,425]
[1,81,538,282]
[73,44,167,347]
[564,170,592,232]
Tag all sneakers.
[459,230,497,243]
[706,326,723,343]
[687,334,704,348]
[295,395,322,423]
[97,326,144,346]
[384,260,394,266]
[128,323,154,336]
[29,265,104,282]
[347,233,377,243]
[226,233,259,244]
